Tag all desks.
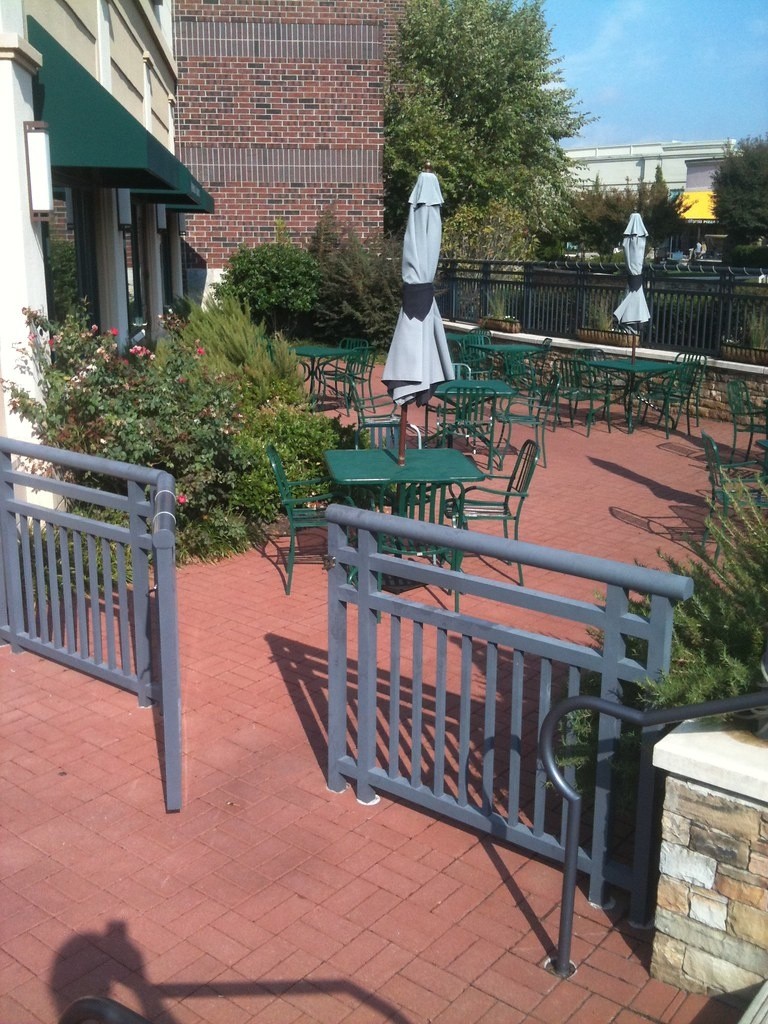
[444,332,485,342]
[756,439,768,449]
[317,449,484,593]
[289,346,356,417]
[469,345,541,398]
[585,359,676,436]
[431,380,513,471]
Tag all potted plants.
[721,313,768,366]
[580,295,640,347]
[479,291,520,333]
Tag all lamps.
[23,121,53,224]
[178,213,186,236]
[156,204,167,233]
[116,189,133,232]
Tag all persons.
[697,241,707,259]
[694,240,702,259]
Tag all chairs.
[265,329,708,623]
[701,430,768,566]
[727,380,768,473]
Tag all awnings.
[674,191,721,225]
[27,14,214,214]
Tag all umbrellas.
[381,173,455,465]
[612,213,651,365]
